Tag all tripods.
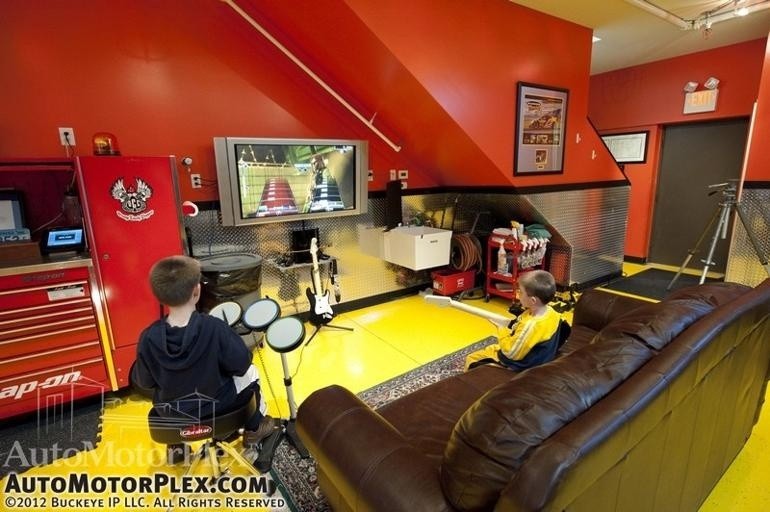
[663,197,770,290]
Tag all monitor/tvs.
[48,225,84,253]
[213,136,369,227]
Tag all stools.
[149,390,277,498]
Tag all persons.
[129,254,290,463]
[464,269,564,371]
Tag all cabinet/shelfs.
[486,228,547,305]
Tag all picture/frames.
[513,82,570,176]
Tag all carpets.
[601,267,725,300]
[257,335,498,511]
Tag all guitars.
[305,237,335,325]
[424,294,571,350]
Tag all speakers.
[292,228,319,264]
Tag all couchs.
[295,277,769,512]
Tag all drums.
[241,297,280,331]
[208,300,242,329]
[265,316,306,353]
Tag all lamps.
[681,77,720,115]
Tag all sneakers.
[167,444,192,464]
[243,415,274,448]
